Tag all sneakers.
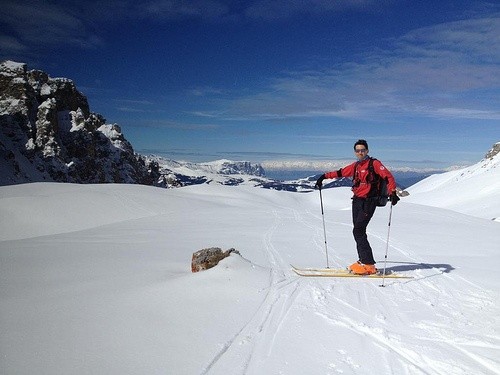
[353,265,376,274]
[347,260,361,270]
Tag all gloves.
[393,190,400,205]
[314,174,327,190]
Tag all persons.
[317,140,399,275]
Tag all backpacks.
[355,157,388,206]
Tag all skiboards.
[289,263,380,274]
[291,269,414,278]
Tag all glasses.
[355,148,367,153]
